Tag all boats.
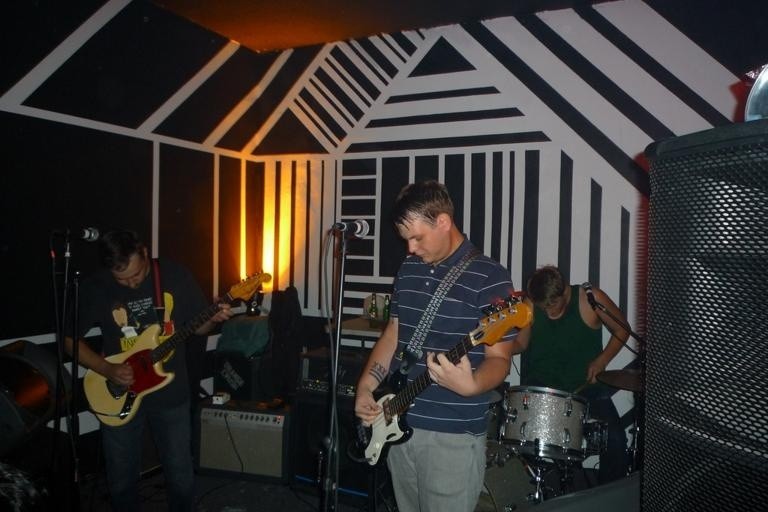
[639,118,767,512]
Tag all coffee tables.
[596,371,645,391]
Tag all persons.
[354,178,514,511]
[64,227,232,510]
[502,263,632,482]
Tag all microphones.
[298,344,339,385]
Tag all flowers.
[332,219,370,239]
[58,227,99,242]
[582,282,597,312]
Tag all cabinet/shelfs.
[381,294,394,327]
[246,272,263,316]
[366,292,380,329]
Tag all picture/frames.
[325,315,388,355]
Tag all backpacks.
[475,441,543,512]
[1,340,55,441]
[502,386,591,461]
